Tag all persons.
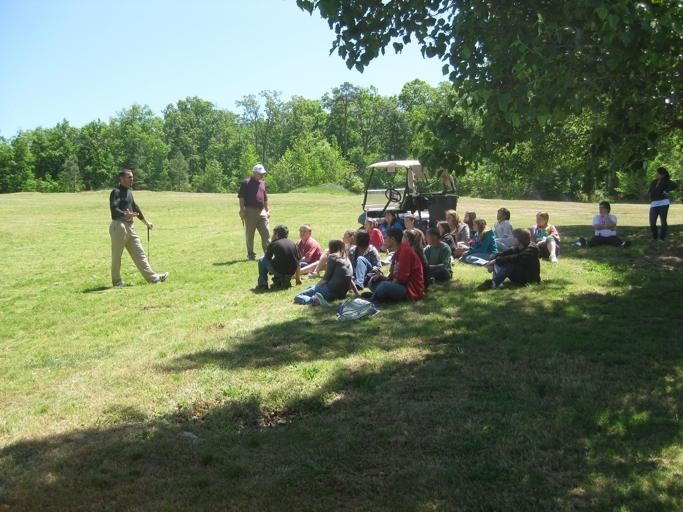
[438,169,457,193]
[256,207,561,307]
[237,164,274,260]
[577,200,632,248]
[109,168,170,289]
[647,167,677,241]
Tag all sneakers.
[621,240,631,247]
[313,292,327,305]
[154,273,168,282]
[548,255,557,264]
[579,238,586,248]
[479,279,493,290]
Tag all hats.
[252,165,266,174]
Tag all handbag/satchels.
[336,298,375,321]
